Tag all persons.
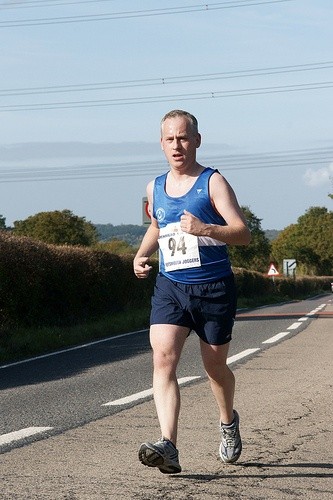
[133,109,249,475]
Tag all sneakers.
[219,409,242,462]
[138,437,182,474]
